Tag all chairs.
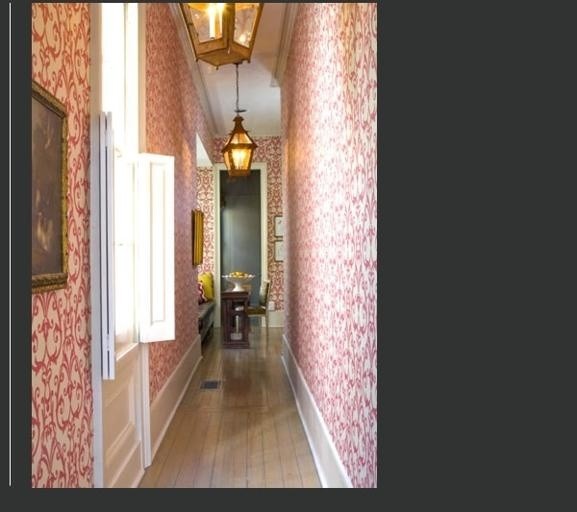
[245,280,271,345]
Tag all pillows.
[197,283,208,304]
[198,272,215,299]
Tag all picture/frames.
[274,214,284,239]
[273,240,285,263]
[31,82,70,295]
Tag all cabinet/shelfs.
[196,300,214,352]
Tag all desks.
[220,288,252,347]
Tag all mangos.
[231,271,243,276]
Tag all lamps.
[221,65,259,179]
[178,2,266,72]
